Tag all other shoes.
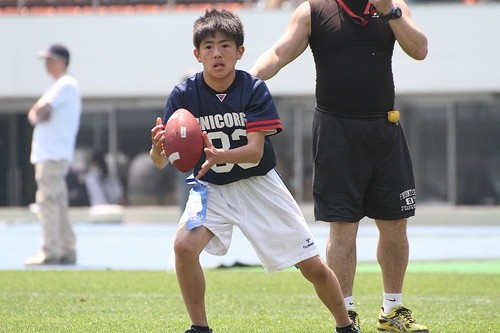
[335,328,361,333]
[24,248,77,265]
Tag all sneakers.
[348,308,360,328]
[377,306,429,333]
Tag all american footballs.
[163,108,204,173]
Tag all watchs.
[384,7,402,22]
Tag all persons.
[150,10,361,333]
[247,0,430,333]
[27,43,77,266]
[86,151,127,206]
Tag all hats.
[36,44,69,66]
